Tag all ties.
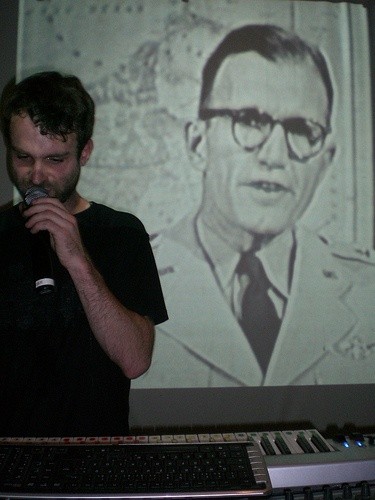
[235,254,282,375]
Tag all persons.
[1,71,169,436]
[129,24,374,384]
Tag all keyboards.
[0,442,274,500]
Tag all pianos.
[0,420,374,500]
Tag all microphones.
[25,185,54,294]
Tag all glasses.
[199,107,328,163]
